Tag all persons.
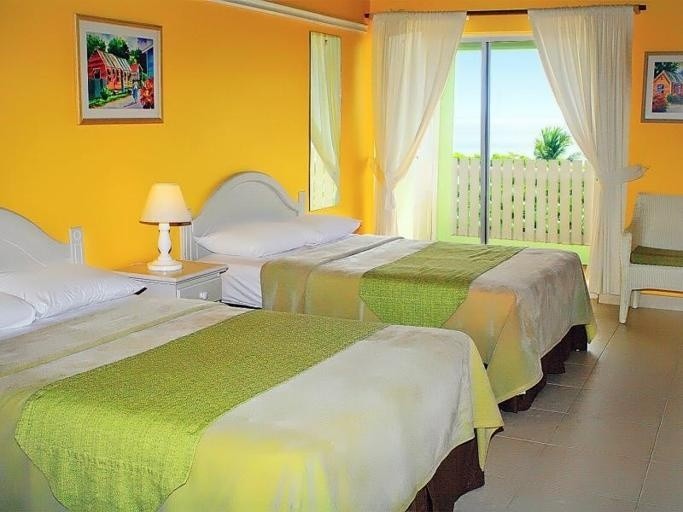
[131,78,140,104]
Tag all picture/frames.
[640,51,683,124]
[73,13,164,126]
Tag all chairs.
[618,192,683,324]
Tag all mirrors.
[308,30,342,213]
